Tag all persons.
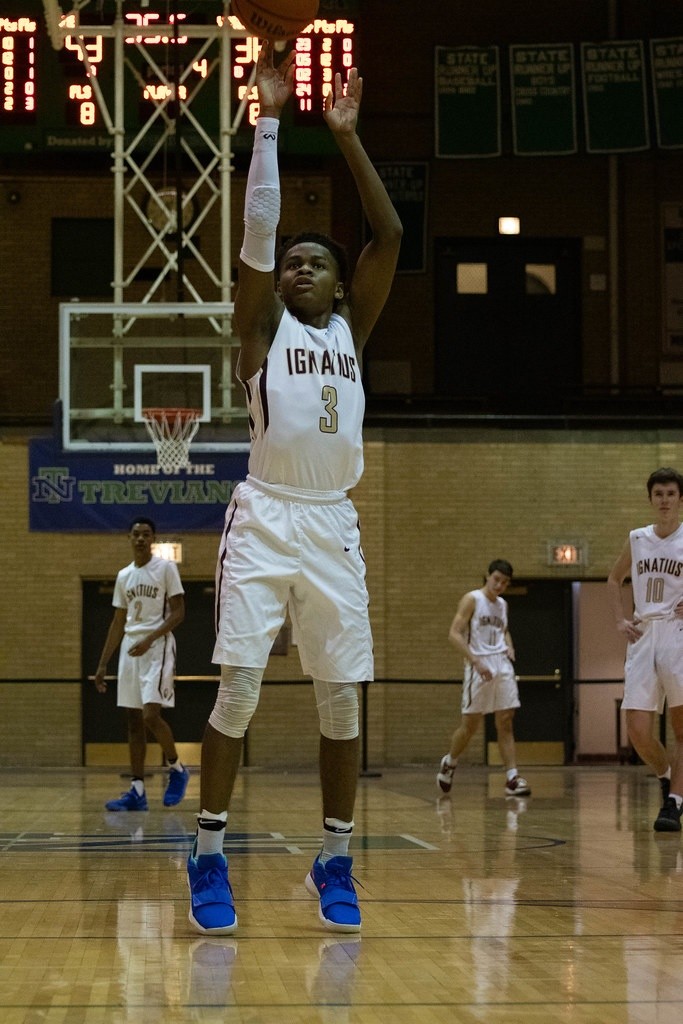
[608,468,683,830]
[94,517,189,812]
[624,831,683,1024]
[438,796,533,1024]
[188,39,403,936]
[437,559,532,796]
[101,813,183,1024]
[188,933,362,1024]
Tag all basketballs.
[230,0,320,41]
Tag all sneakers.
[105,786,149,812]
[162,768,190,807]
[658,777,671,797]
[504,776,531,795]
[186,835,237,935]
[305,852,361,934]
[436,755,456,793]
[653,797,683,832]
